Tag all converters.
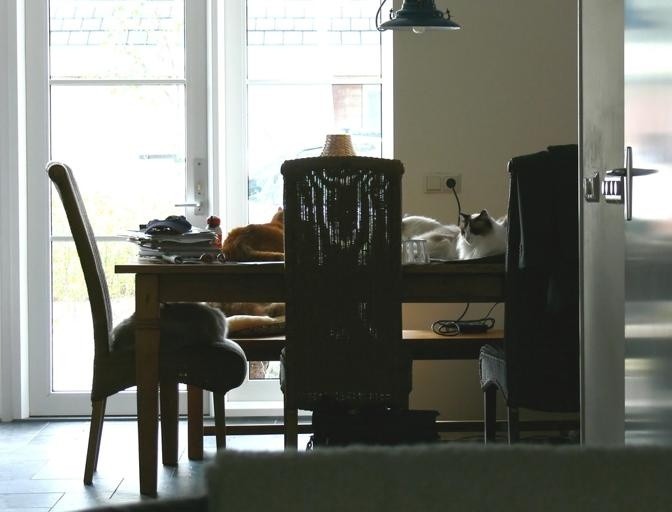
[457,323,488,334]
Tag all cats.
[401,209,509,259]
[223,206,284,262]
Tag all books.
[129,227,217,265]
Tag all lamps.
[315,133,358,158]
[374,1,463,37]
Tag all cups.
[403,239,430,266]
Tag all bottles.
[205,216,223,253]
